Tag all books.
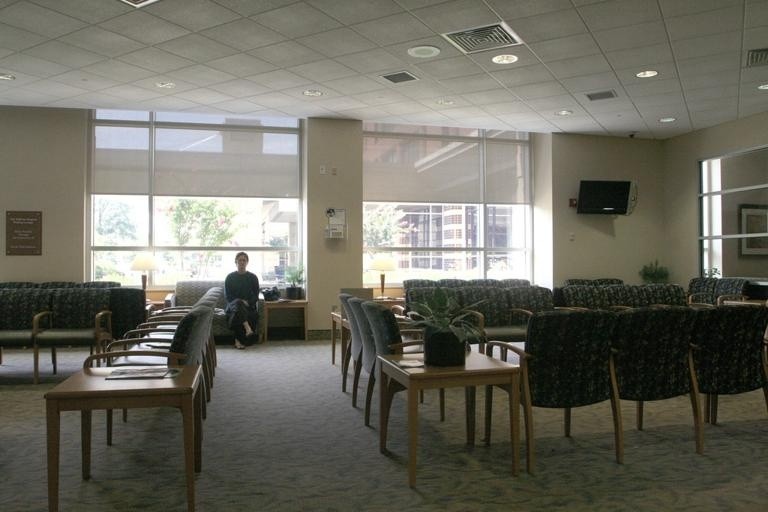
[105,367,170,377]
[163,367,185,378]
[389,357,425,369]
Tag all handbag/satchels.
[261,287,280,301]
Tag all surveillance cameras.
[628,132,635,138]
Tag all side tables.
[43,364,202,511]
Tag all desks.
[330,311,348,374]
[264,299,309,342]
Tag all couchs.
[165,281,265,344]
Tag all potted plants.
[284,266,303,300]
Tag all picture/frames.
[5,210,42,256]
[737,203,768,258]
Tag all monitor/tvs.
[577,180,630,214]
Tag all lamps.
[130,252,160,289]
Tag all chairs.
[0,281,223,445]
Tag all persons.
[223,251,260,349]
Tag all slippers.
[235,333,255,349]
